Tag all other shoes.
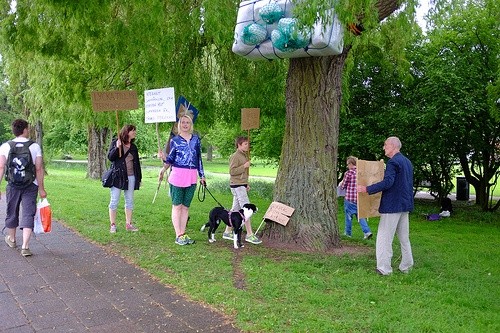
[22,248,32,256]
[364,231,373,239]
[372,268,383,275]
[5,235,16,248]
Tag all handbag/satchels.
[101,171,115,187]
[34,197,51,234]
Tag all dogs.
[201,203,258,249]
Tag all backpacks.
[5,140,36,187]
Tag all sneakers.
[126,222,138,231]
[245,233,263,245]
[110,223,116,233]
[222,230,234,240]
[175,234,195,245]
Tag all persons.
[0,120,47,257]
[101,125,142,232]
[223,136,263,245]
[356,138,414,276]
[338,155,373,240]
[158,115,207,245]
